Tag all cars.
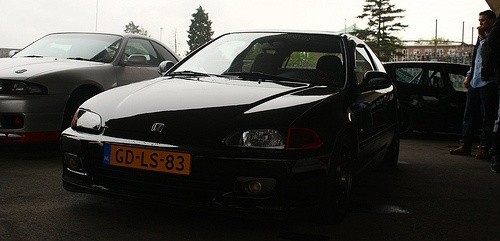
[60,30,403,228]
[382,61,472,140]
[0,32,181,157]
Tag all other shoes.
[474,148,489,160]
[449,146,472,155]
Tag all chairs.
[432,76,445,89]
[315,56,342,84]
[251,52,280,75]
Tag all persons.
[449,10,500,159]
